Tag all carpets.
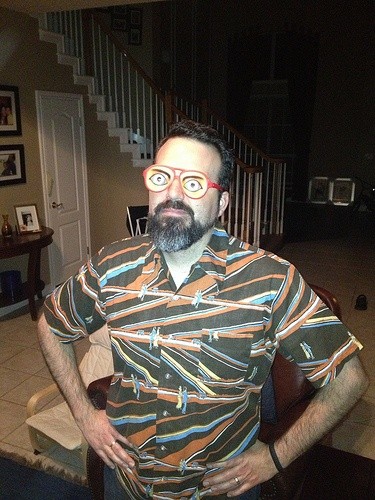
[0,449,92,500]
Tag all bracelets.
[270,440,283,472]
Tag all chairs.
[26,323,114,474]
[86,284,342,500]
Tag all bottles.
[1,214,12,239]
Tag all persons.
[0,106,13,125]
[36,119,370,500]
[1,154,16,176]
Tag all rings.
[234,477,241,487]
[110,442,116,447]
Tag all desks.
[0,225,54,320]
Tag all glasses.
[142,164,224,199]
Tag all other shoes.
[355,294,367,310]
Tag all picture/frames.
[14,203,44,235]
[0,144,26,185]
[0,85,22,136]
